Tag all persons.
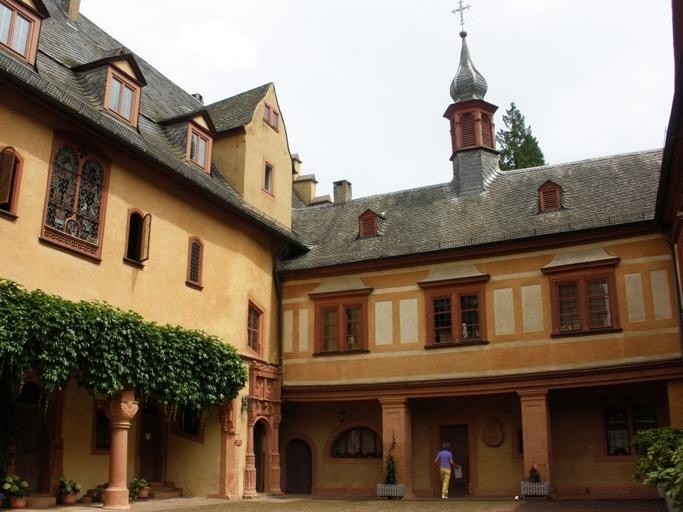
[432,442,458,501]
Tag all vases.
[9,496,25,508]
[62,492,76,504]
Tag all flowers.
[3,474,28,498]
[57,476,81,494]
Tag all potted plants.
[137,479,149,498]
[632,441,683,512]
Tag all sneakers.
[442,495,448,498]
